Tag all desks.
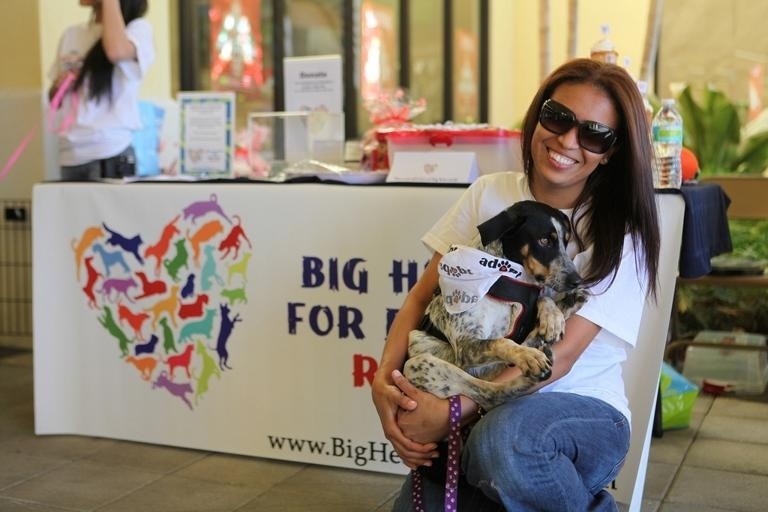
[29,175,732,510]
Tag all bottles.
[636,80,682,190]
[590,22,617,65]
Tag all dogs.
[400,200,594,412]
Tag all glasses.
[538,99,617,154]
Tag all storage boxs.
[376,128,523,183]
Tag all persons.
[48,1,154,181]
[372,57,660,512]
